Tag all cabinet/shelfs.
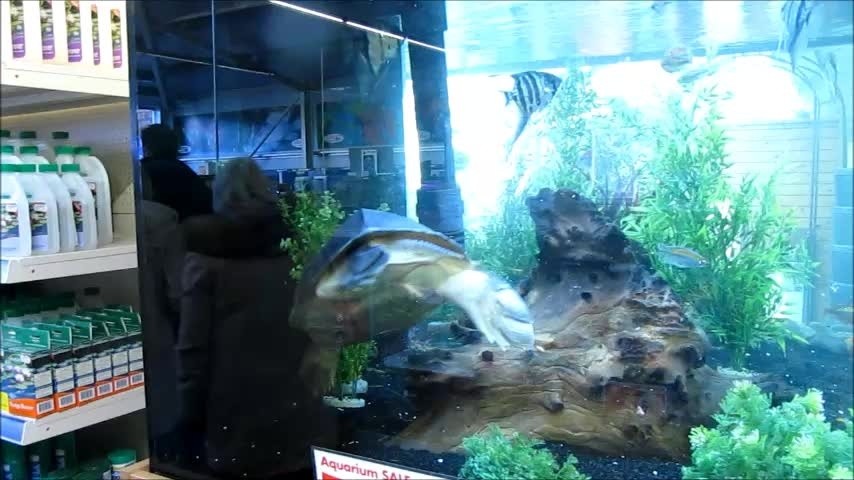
[0,0,146,446]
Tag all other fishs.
[650,45,694,74]
[780,0,818,75]
[657,243,709,269]
[504,70,562,115]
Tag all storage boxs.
[123,0,854,480]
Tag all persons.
[134,122,305,480]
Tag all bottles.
[73,146,113,245]
[0,164,32,257]
[0,437,137,480]
[20,130,57,163]
[18,146,49,173]
[53,145,74,177]
[78,287,104,307]
[55,291,78,310]
[48,131,76,158]
[17,164,60,254]
[39,162,78,253]
[0,130,20,159]
[61,163,98,250]
[0,145,23,164]
